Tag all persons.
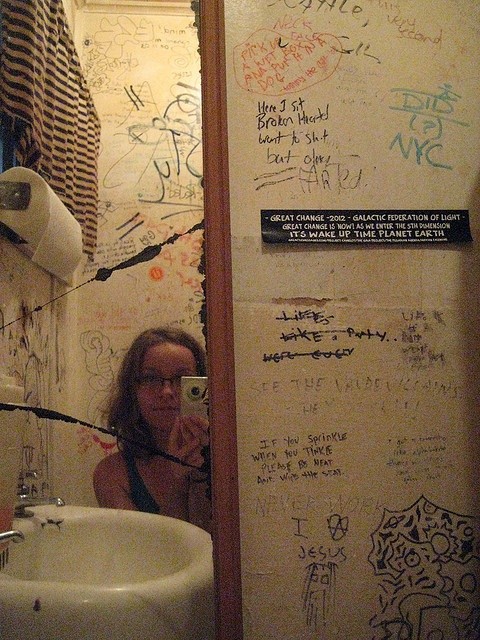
[93,324,213,534]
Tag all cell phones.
[180,375,210,419]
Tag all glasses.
[136,370,194,386]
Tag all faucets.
[4,466,66,519]
[0,527,26,563]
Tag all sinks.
[3,514,199,589]
